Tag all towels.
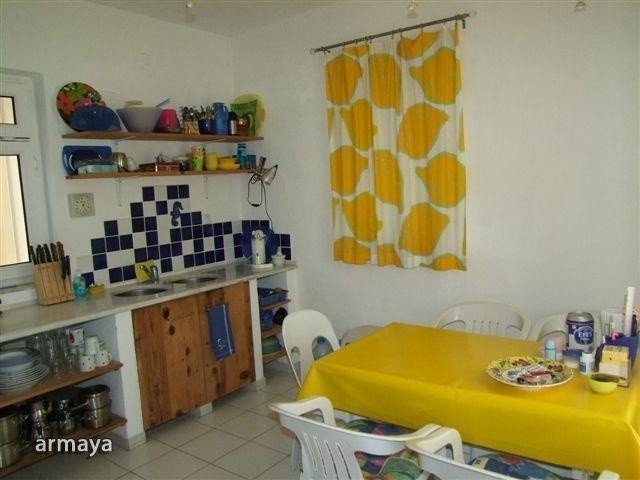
[206,301,237,361]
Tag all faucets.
[139,265,159,284]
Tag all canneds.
[566,312,594,354]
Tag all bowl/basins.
[271,255,286,267]
[589,372,617,393]
[114,108,162,132]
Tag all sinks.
[111,286,168,298]
[167,274,219,286]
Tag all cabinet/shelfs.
[129,278,260,434]
[257,267,295,367]
[60,128,267,181]
[0,310,129,479]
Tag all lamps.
[180,2,196,22]
[574,1,588,14]
[406,0,418,20]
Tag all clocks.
[67,191,96,219]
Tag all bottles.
[545,340,556,361]
[237,143,249,168]
[191,146,204,171]
[255,254,260,265]
[228,112,238,136]
[579,352,594,376]
[72,270,87,294]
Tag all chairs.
[267,391,444,480]
[426,302,532,341]
[280,308,370,478]
[530,313,603,350]
[404,425,621,480]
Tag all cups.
[205,153,240,170]
[248,155,256,169]
[30,327,111,378]
[184,119,199,136]
[200,119,214,134]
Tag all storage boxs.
[258,287,289,305]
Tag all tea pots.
[213,103,228,135]
[159,109,181,133]
[112,152,138,172]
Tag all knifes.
[27,240,72,282]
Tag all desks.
[293,320,638,479]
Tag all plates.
[0,347,50,395]
[486,355,573,391]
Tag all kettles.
[251,231,267,263]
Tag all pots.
[0,383,113,469]
